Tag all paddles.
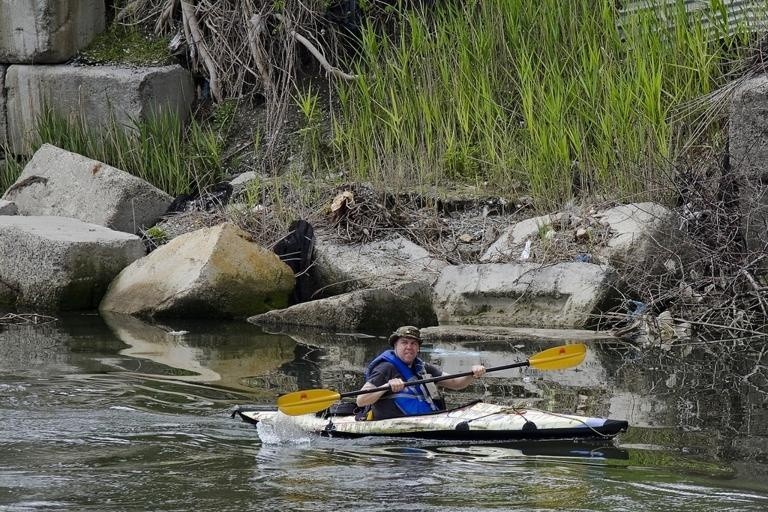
[278,343,587,417]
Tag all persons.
[356,325,487,421]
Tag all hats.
[388,326,423,347]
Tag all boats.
[231,400,628,445]
